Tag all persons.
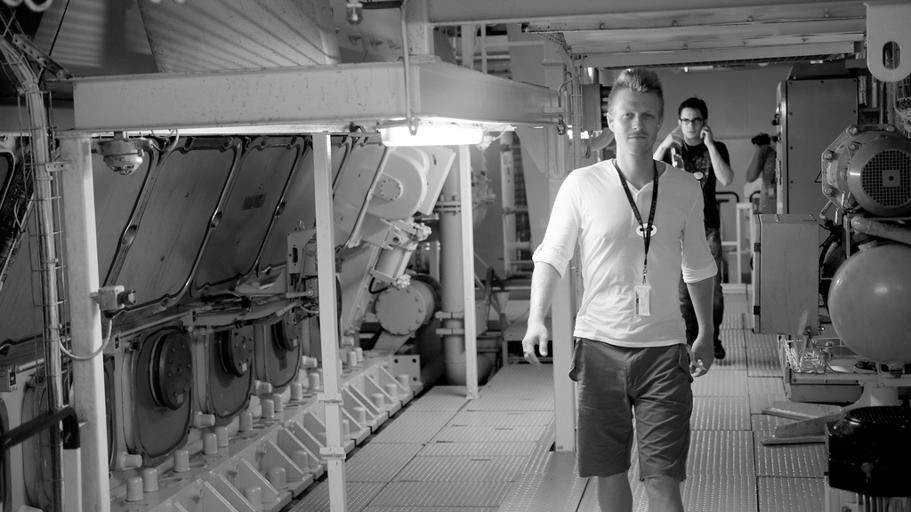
[520,67,720,511]
[743,133,778,217]
[655,95,736,361]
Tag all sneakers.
[715,341,725,359]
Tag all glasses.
[678,118,704,124]
